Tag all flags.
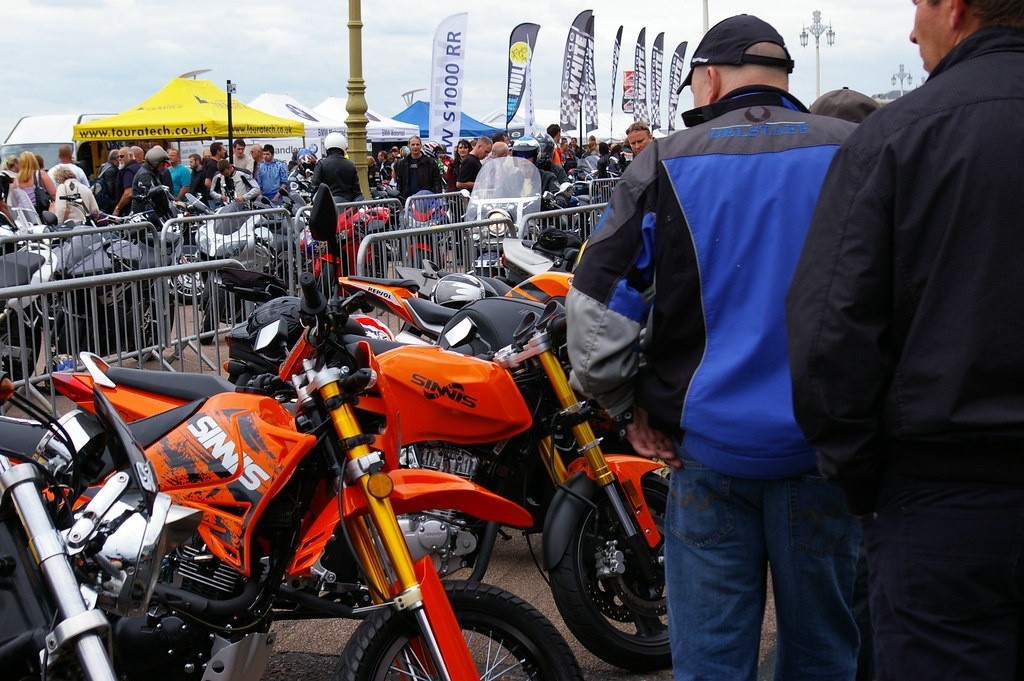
[429,10,688,158]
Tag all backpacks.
[90,164,113,204]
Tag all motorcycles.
[1,142,680,681]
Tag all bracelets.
[116,205,122,211]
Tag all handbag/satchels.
[33,169,50,212]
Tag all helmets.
[247,296,301,342]
[512,137,541,169]
[324,131,347,154]
[422,141,443,159]
[429,272,486,309]
[145,147,171,168]
[536,133,556,162]
[297,148,317,164]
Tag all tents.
[303,98,420,143]
[390,100,504,141]
[72,77,306,164]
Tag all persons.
[0,122,658,259]
[565,15,882,681]
[785,0,1024,681]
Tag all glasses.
[117,154,128,158]
[516,153,533,159]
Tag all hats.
[810,86,879,124]
[677,14,794,95]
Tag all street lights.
[891,64,913,97]
[799,10,836,100]
